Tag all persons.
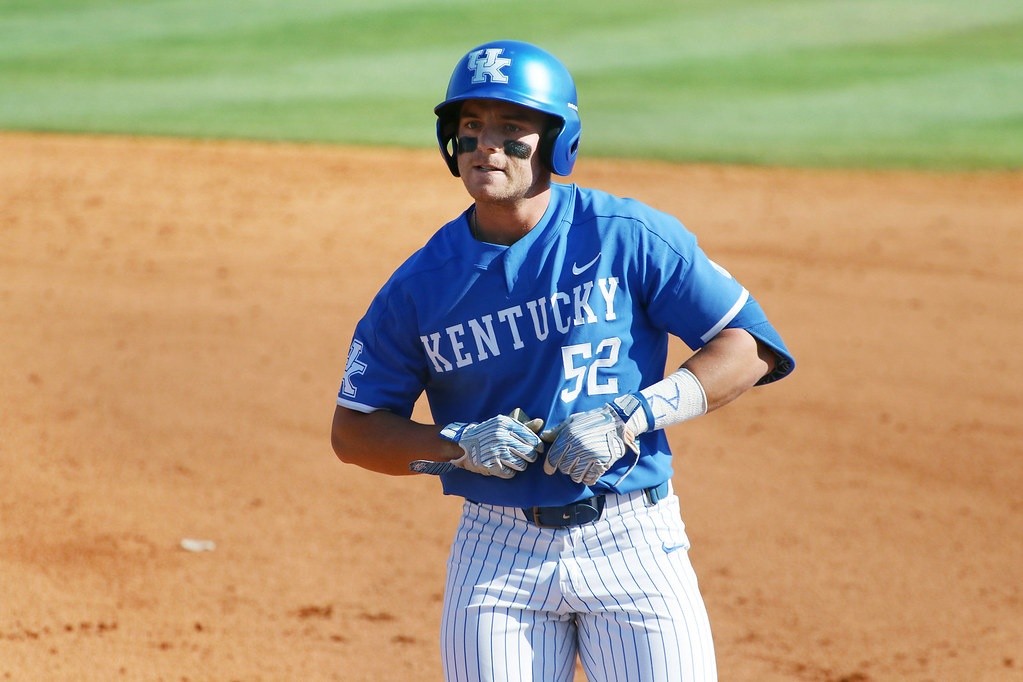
[331,40,795,682]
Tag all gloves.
[408,414,544,479]
[539,394,641,488]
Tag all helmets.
[434,40,580,176]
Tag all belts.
[467,487,653,528]
